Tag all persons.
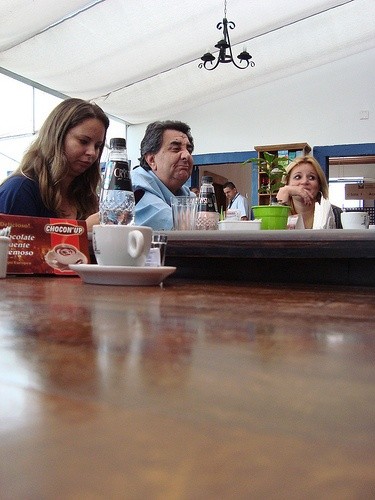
[130,120,197,232]
[189,185,199,198]
[0,98,133,232]
[222,182,249,222]
[270,156,343,229]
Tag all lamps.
[198,0,256,70]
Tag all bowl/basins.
[340,212,370,230]
[217,220,262,231]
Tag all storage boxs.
[0,213,90,275]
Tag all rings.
[305,196,307,198]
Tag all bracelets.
[277,199,286,205]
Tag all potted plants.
[240,152,293,230]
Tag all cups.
[170,196,198,232]
[0,235,11,278]
[53,244,78,265]
[92,224,153,267]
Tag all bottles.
[196,176,222,231]
[261,177,266,194]
[99,138,139,225]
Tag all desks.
[163,228,375,287]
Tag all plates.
[45,249,88,272]
[68,264,176,287]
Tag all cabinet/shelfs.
[254,143,311,205]
